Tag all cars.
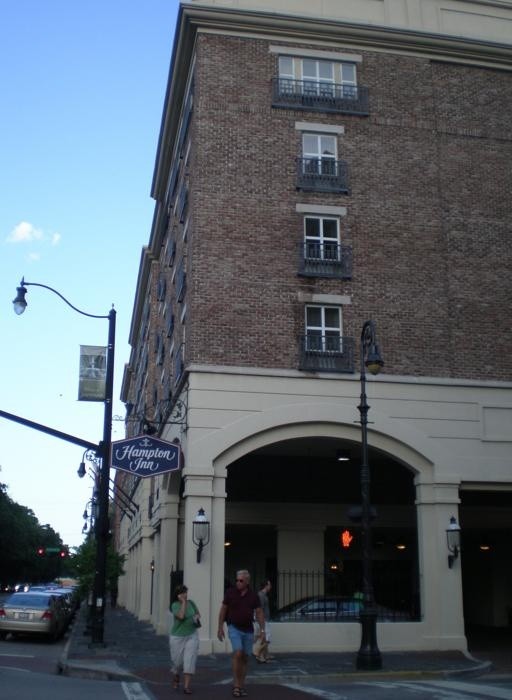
[273,595,414,622]
[0,583,85,644]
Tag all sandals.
[173,679,191,695]
[232,686,247,697]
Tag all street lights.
[15,282,115,644]
[358,321,384,671]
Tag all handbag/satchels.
[193,619,201,628]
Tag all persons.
[218,569,265,697]
[169,584,200,694]
[255,578,272,663]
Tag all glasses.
[235,579,244,583]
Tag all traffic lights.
[58,546,68,560]
[36,545,45,558]
[336,527,362,555]
